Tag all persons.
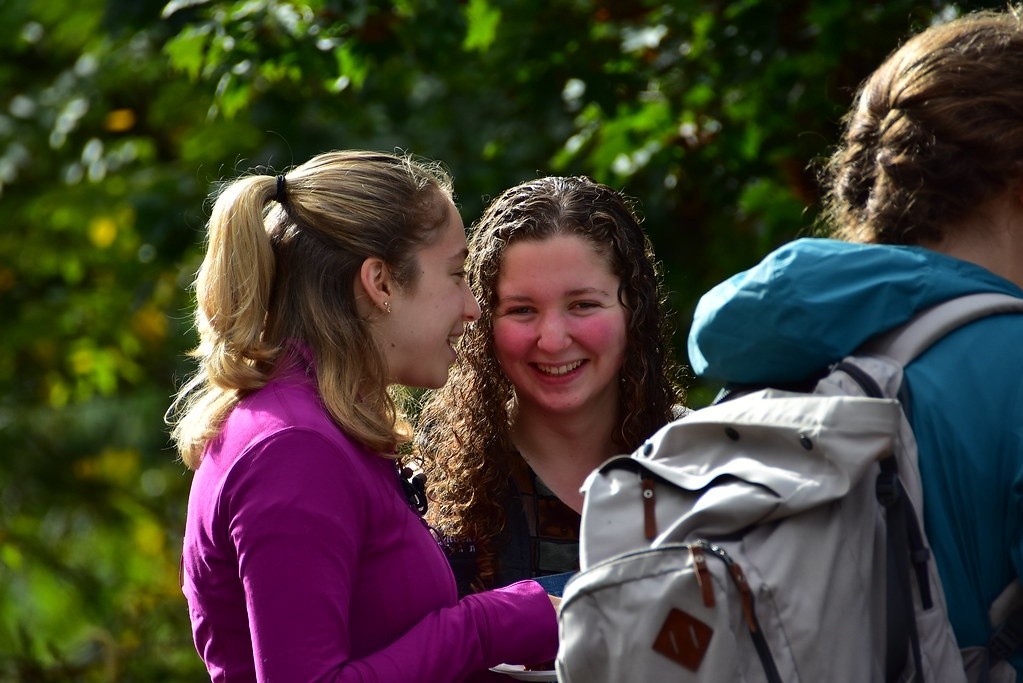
[686,4,1023,683]
[166,147,562,683]
[397,175,701,603]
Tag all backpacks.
[554,292,1023,682]
